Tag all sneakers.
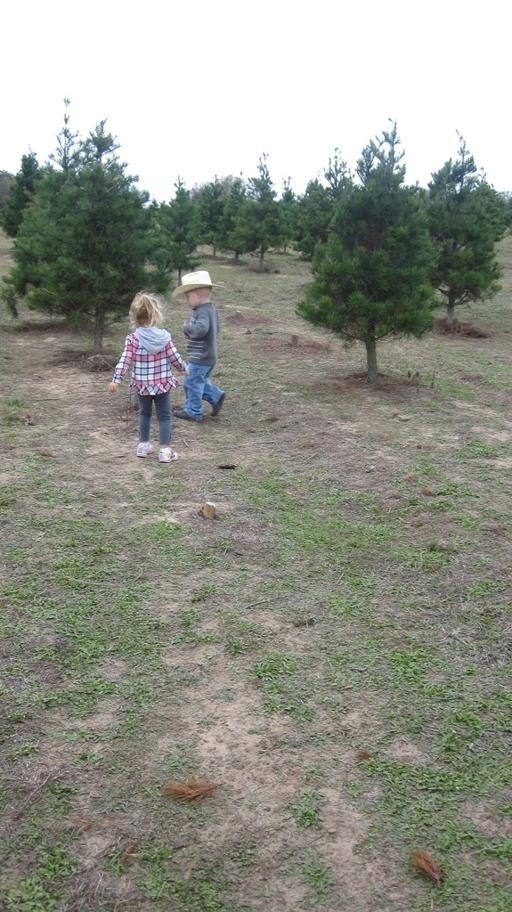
[159,447,178,463]
[137,441,154,458]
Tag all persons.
[110,288,192,464]
[172,270,228,423]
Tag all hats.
[172,270,226,297]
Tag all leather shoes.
[173,409,194,421]
[211,393,226,416]
